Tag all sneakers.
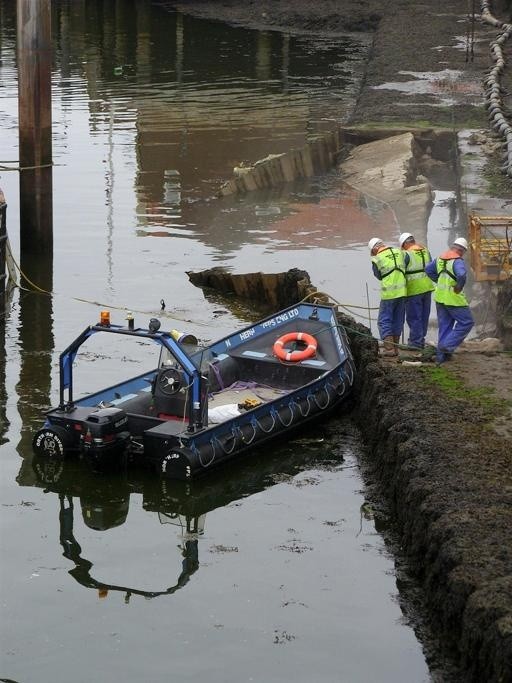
[440,344,449,357]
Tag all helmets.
[454,238,468,252]
[368,237,382,256]
[399,232,413,249]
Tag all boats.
[30,300,358,485]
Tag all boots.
[378,335,399,357]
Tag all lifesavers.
[273,332,318,361]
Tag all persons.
[399,233,432,349]
[425,237,475,361]
[369,238,411,357]
